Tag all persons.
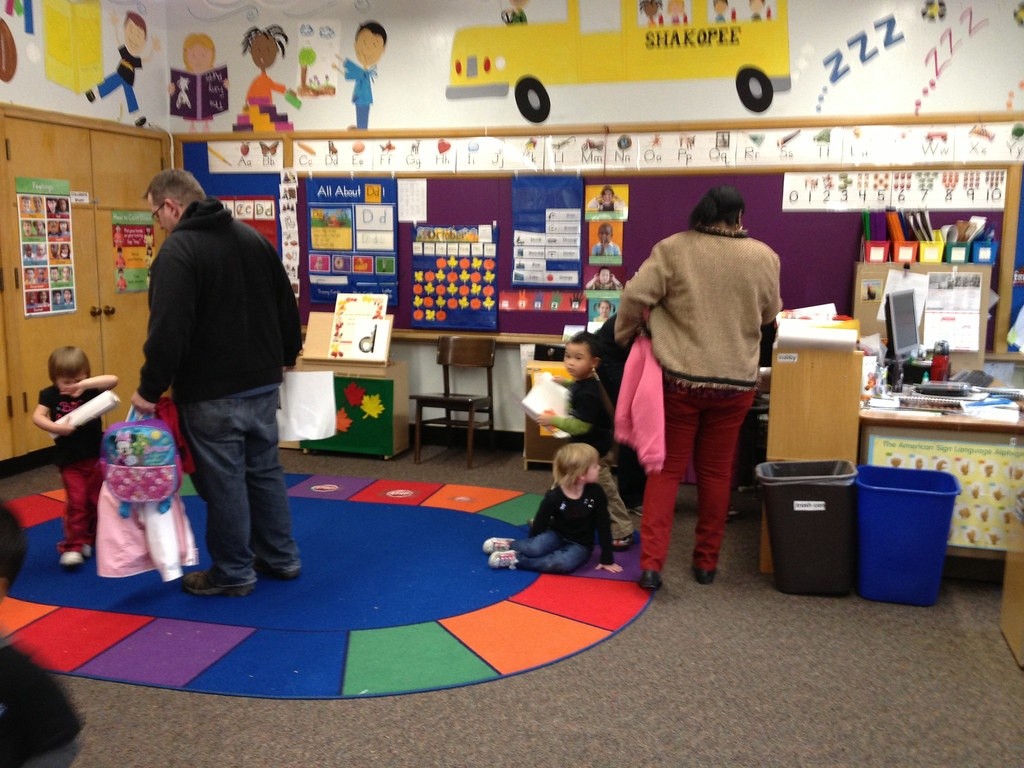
[587,185,626,211]
[613,184,784,588]
[20,197,74,309]
[585,266,624,291]
[482,442,624,575]
[32,346,119,569]
[592,300,615,323]
[144,225,154,288]
[0,499,84,768]
[114,223,128,293]
[535,330,635,552]
[130,168,302,596]
[595,313,647,518]
[591,222,621,257]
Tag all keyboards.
[959,370,993,387]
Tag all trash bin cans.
[756,459,859,599]
[854,465,960,608]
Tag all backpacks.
[100,420,184,518]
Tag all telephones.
[914,380,970,396]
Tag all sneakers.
[482,537,516,554]
[183,570,257,596]
[488,549,519,569]
[254,560,302,581]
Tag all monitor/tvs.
[882,290,919,363]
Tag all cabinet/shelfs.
[299,312,409,459]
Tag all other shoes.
[693,564,717,585]
[639,570,661,588]
[527,517,534,528]
[59,551,83,565]
[726,505,740,522]
[82,544,92,557]
[628,505,643,516]
[612,535,635,551]
[737,479,763,492]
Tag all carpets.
[0,471,656,700]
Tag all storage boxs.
[894,241,917,264]
[865,240,891,262]
[974,239,999,265]
[919,240,946,264]
[947,243,971,264]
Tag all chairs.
[409,336,497,468]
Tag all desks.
[761,343,864,574]
[523,359,569,469]
[862,375,1024,561]
